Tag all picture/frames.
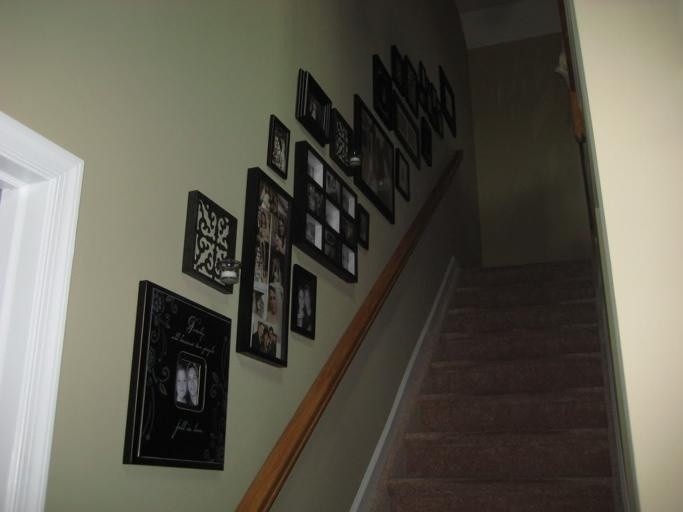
[233,159,295,371]
[180,189,239,294]
[265,41,457,284]
[123,279,233,471]
[290,263,317,340]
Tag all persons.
[173,136,312,409]
